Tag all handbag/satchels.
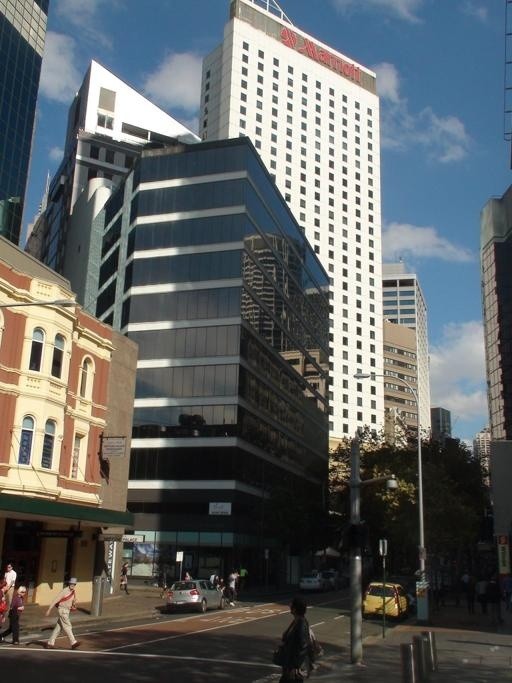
[308,627,325,661]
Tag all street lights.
[350,443,398,666]
[0,298,78,307]
[353,373,431,621]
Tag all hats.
[68,578,77,584]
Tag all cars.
[299,567,351,593]
[166,579,226,613]
[362,582,409,619]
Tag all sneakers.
[45,645,58,649]
[72,641,82,650]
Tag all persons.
[122,560,132,595]
[0,581,8,642]
[429,567,512,625]
[44,577,83,649]
[271,594,315,682]
[184,569,242,607]
[0,584,28,646]
[3,562,17,613]
